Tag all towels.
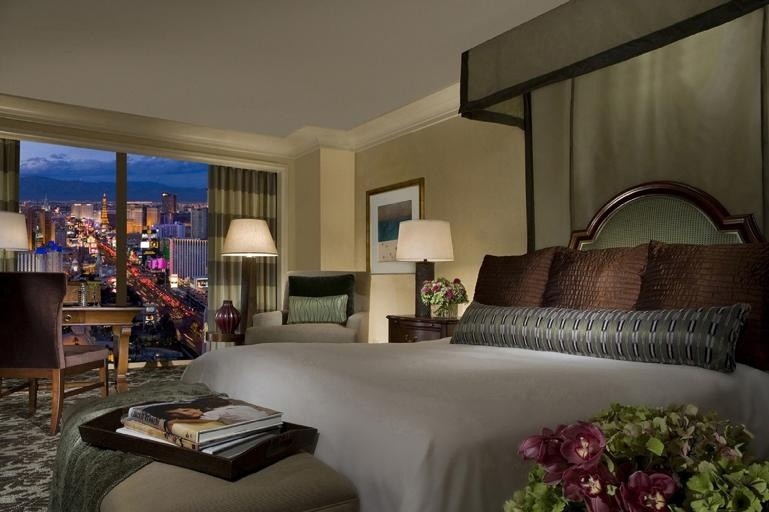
[49,380,228,512]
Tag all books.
[115,394,285,459]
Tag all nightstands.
[386,315,457,342]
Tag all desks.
[61,305,146,394]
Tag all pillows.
[287,295,349,323]
[288,274,355,327]
[638,240,769,373]
[541,243,649,315]
[474,247,556,308]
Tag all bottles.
[80,283,88,307]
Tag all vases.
[434,304,457,318]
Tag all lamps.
[219,218,279,331]
[0,211,30,271]
[395,219,454,317]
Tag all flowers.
[421,277,469,320]
[504,404,769,512]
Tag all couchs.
[244,271,371,344]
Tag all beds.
[179,181,769,511]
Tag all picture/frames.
[366,177,425,275]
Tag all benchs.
[51,379,359,511]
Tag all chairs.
[0,271,111,435]
